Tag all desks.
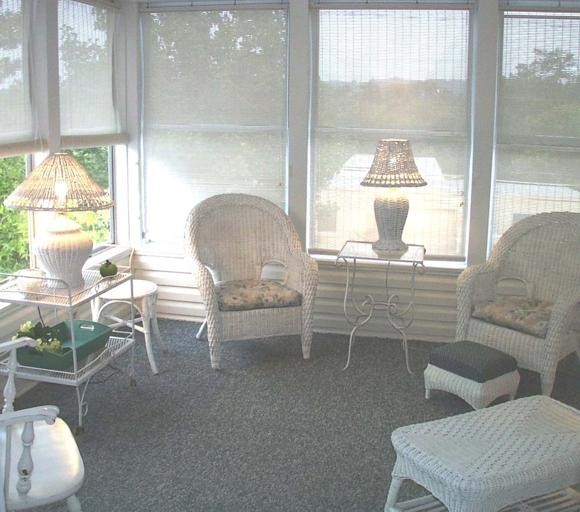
[333,238,427,377]
[89,280,171,377]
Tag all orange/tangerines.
[100,262,117,277]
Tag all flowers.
[15,317,67,356]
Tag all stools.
[419,342,522,411]
[383,392,580,512]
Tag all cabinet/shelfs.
[1,241,138,434]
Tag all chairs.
[0,334,89,512]
[451,209,580,402]
[188,191,322,372]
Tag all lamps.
[1,151,117,291]
[358,137,426,251]
